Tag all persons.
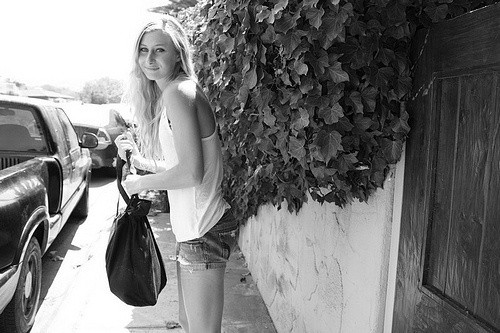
[114,16,237,333]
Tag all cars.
[60,102,133,179]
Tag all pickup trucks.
[0,92,100,330]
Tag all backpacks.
[105,147,167,307]
